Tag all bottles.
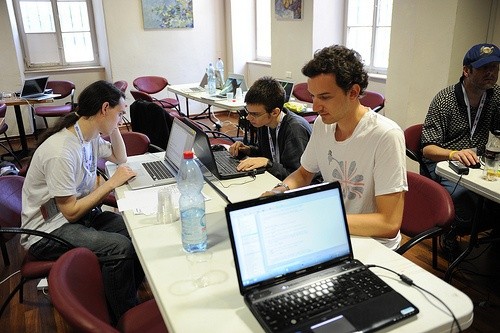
[216,58,225,88]
[207,64,216,94]
[176,151,209,252]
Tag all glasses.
[244,105,270,118]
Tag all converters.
[449,160,469,175]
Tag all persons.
[21,79,145,318]
[260,44,409,251]
[228,76,323,187]
[420,43,500,253]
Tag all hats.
[463,43,500,68]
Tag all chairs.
[0,76,476,333]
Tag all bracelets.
[449,151,457,160]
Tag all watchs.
[275,182,290,191]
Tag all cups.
[235,88,242,102]
[227,93,233,102]
[484,159,500,181]
[155,188,179,223]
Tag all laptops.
[117,116,196,188]
[20,76,62,101]
[183,117,268,182]
[225,181,420,333]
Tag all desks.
[168,83,248,137]
[105,144,475,333]
[0,94,54,163]
[435,155,500,282]
[285,96,319,124]
